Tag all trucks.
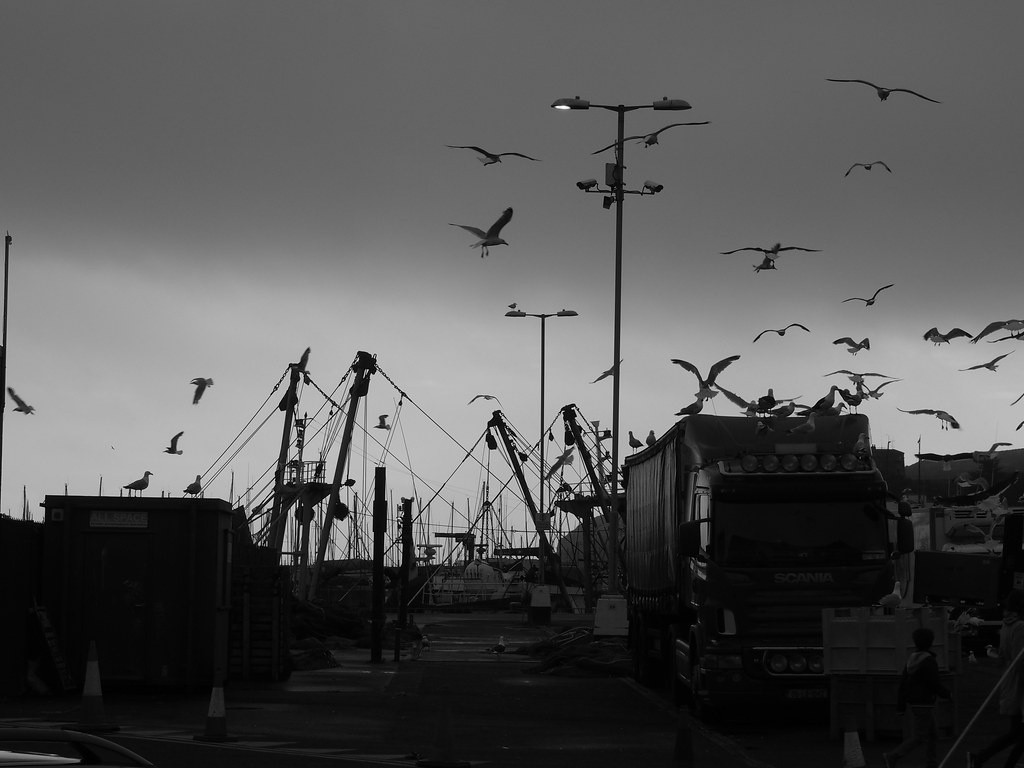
[628,413,916,741]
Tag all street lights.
[551,95,692,639]
[504,308,578,610]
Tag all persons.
[965,589,1024,768]
[881,628,945,768]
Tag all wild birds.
[845,160,891,176]
[589,358,624,384]
[955,607,1000,659]
[123,471,153,498]
[509,302,517,310]
[447,145,542,166]
[543,446,576,480]
[590,122,711,156]
[421,636,431,652]
[885,488,913,503]
[7,386,35,416]
[162,431,184,455]
[720,242,823,273]
[468,396,503,409]
[826,79,941,104]
[183,475,202,499]
[446,207,513,258]
[556,483,574,494]
[111,446,115,450]
[191,377,213,406]
[492,635,506,655]
[628,284,1024,473]
[871,582,903,609]
[966,482,1013,552]
[373,414,390,431]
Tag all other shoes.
[966,752,981,768]
[882,753,894,768]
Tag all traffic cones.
[62,642,122,736]
[190,665,241,744]
[833,711,866,767]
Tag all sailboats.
[177,346,626,614]
